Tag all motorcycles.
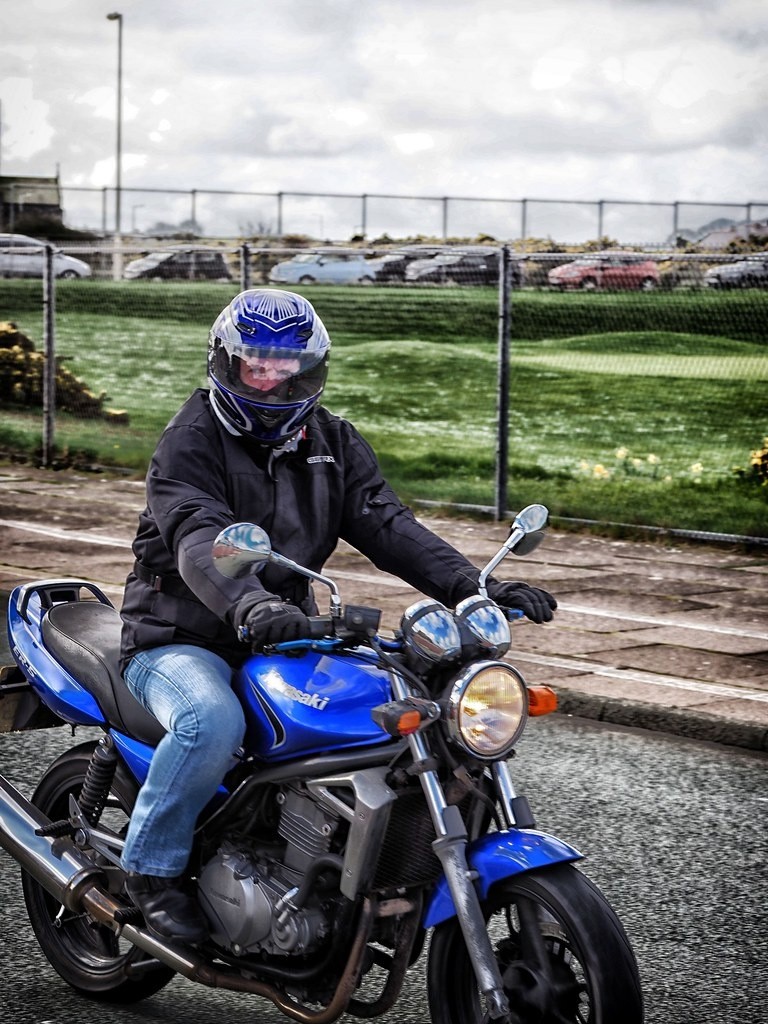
[1,505,647,1024]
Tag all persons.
[118,287,558,944]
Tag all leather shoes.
[123,869,207,944]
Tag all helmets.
[207,288,331,445]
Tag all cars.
[372,243,454,283]
[402,247,524,289]
[0,232,91,281]
[520,257,576,286]
[267,247,377,287]
[548,247,662,293]
[124,245,235,283]
[704,252,768,287]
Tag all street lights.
[106,9,124,282]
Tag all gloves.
[498,587,557,626]
[244,603,310,659]
